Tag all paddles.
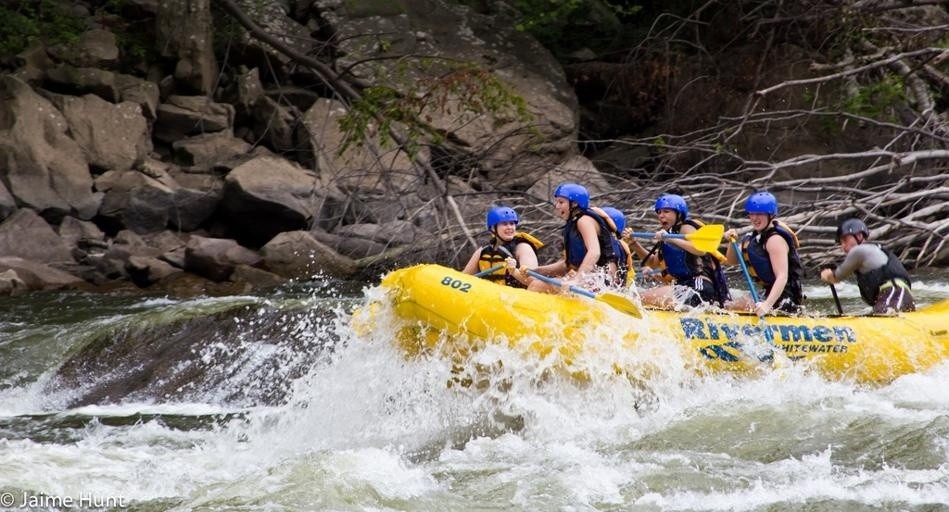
[627,223,726,252]
[518,264,645,321]
[731,238,784,381]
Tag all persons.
[723,192,803,318]
[461,207,539,289]
[820,217,916,315]
[642,219,730,303]
[518,181,617,298]
[600,206,634,289]
[621,194,715,313]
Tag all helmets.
[655,193,689,221]
[487,205,518,230]
[745,191,778,216]
[835,217,869,242]
[600,207,625,234]
[554,182,590,208]
